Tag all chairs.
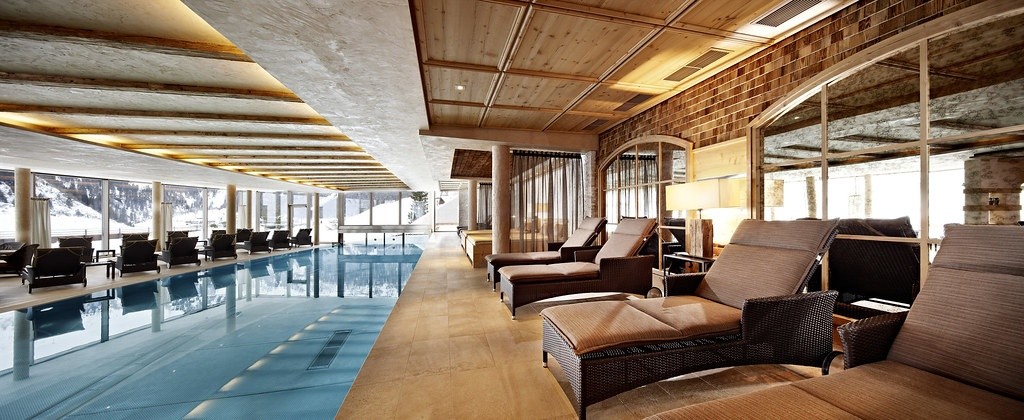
[538,219,839,420]
[497,218,659,319]
[456,215,492,235]
[807,215,930,308]
[485,216,608,292]
[642,222,1024,420]
[0,229,314,294]
